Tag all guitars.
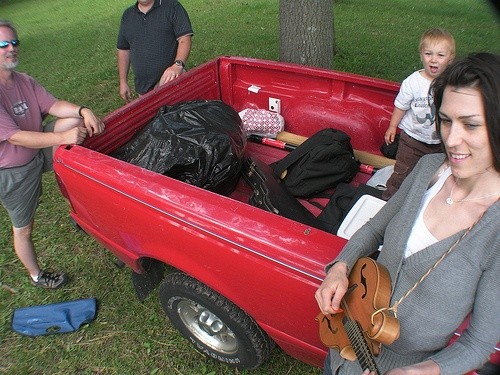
[315,257,400,375]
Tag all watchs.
[173,60,186,68]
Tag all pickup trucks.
[51,54,500,372]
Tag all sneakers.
[28,268,69,290]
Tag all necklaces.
[446,180,498,204]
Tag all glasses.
[0,39,20,48]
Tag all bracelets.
[79,106,91,117]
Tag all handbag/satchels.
[10,295,97,338]
[313,183,381,237]
[380,133,400,159]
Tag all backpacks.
[271,127,360,199]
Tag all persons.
[0,19,105,291]
[381,27,457,202]
[314,51,500,375]
[115,0,194,101]
